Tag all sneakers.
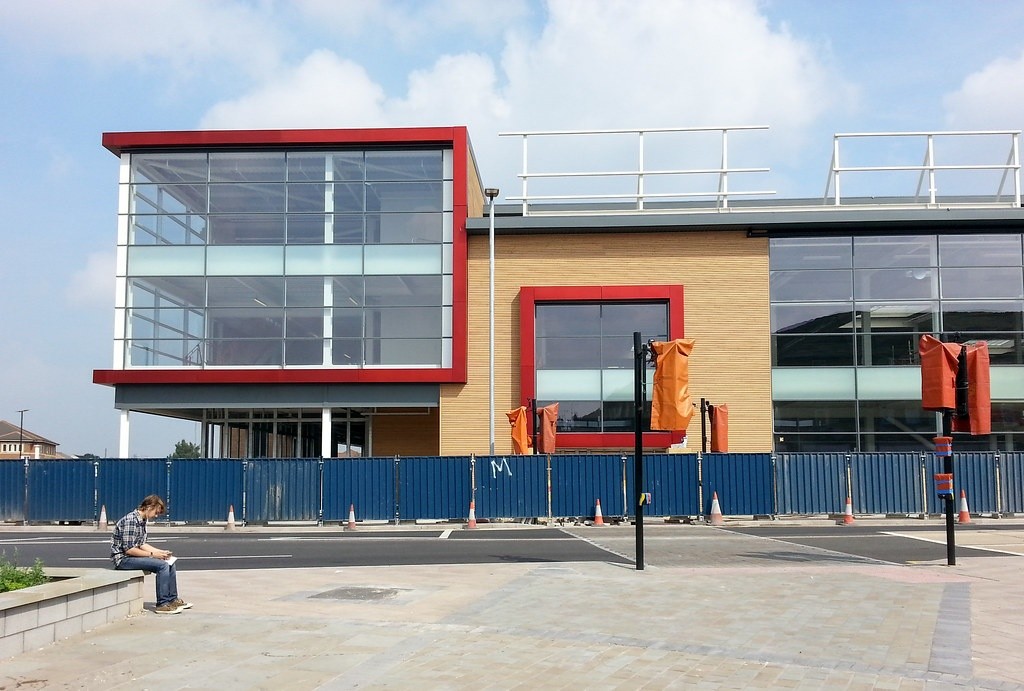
[174,598,192,609]
[155,602,182,614]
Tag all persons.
[110,495,193,614]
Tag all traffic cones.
[96,505,110,531]
[708,491,725,526]
[346,503,358,531]
[223,505,237,531]
[844,497,854,524]
[958,489,972,523]
[466,502,478,528]
[594,499,605,526]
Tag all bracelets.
[149,552,153,558]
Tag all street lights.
[485,187,500,457]
[17,410,29,460]
